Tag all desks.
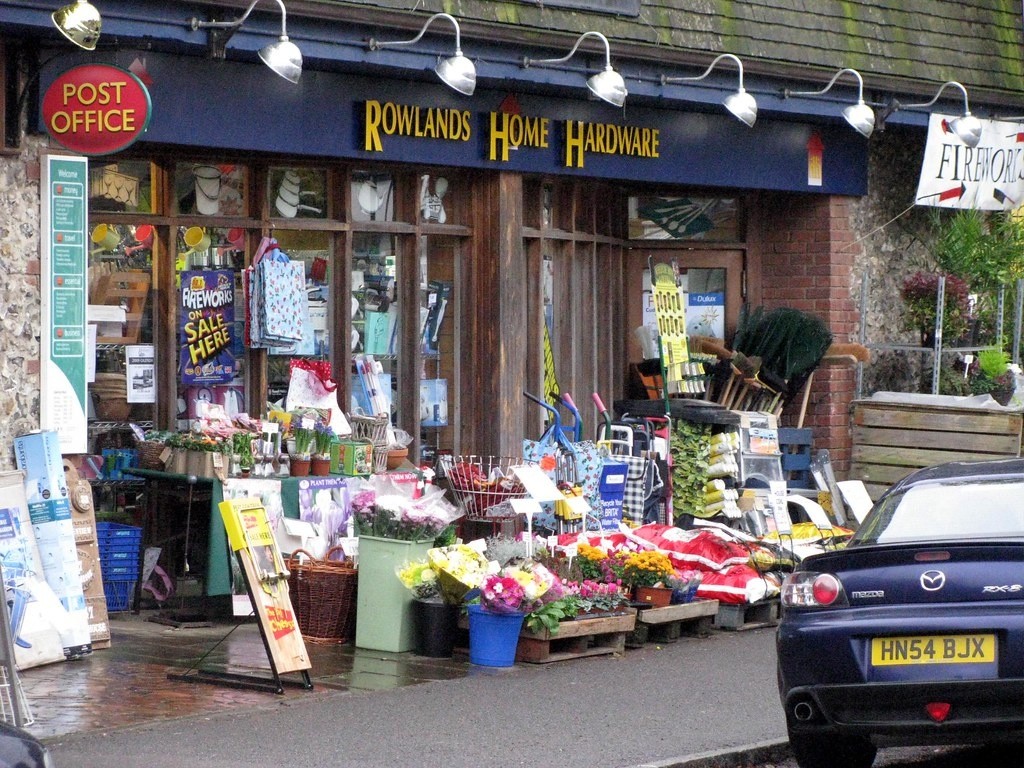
[121,467,426,615]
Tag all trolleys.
[522,386,672,533]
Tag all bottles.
[232,453,288,478]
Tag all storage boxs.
[95,521,143,611]
[330,441,373,475]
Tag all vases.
[411,599,461,658]
[578,594,631,615]
[636,586,674,608]
[355,535,436,652]
[88,373,133,420]
[467,604,525,667]
[186,450,229,478]
[373,447,408,470]
[164,448,188,475]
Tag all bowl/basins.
[386,449,409,470]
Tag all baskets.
[440,455,538,521]
[88,373,132,420]
[282,546,360,646]
[345,407,389,475]
[132,429,166,472]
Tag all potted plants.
[290,427,312,476]
[311,430,333,475]
[896,271,967,347]
[973,342,1016,406]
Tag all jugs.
[178,227,212,261]
[217,228,245,256]
[89,223,121,258]
[124,224,153,256]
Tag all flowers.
[505,562,555,611]
[393,555,442,604]
[427,544,490,604]
[532,543,678,612]
[170,433,218,451]
[347,476,464,540]
[481,576,525,614]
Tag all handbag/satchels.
[522,425,604,529]
[286,358,353,436]
[141,547,174,601]
[241,238,315,354]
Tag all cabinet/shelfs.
[856,272,1006,400]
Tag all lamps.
[192,0,303,84]
[524,31,626,108]
[52,0,102,50]
[898,81,982,149]
[784,68,875,139]
[369,12,476,97]
[660,54,757,128]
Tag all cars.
[774,455,1024,768]
